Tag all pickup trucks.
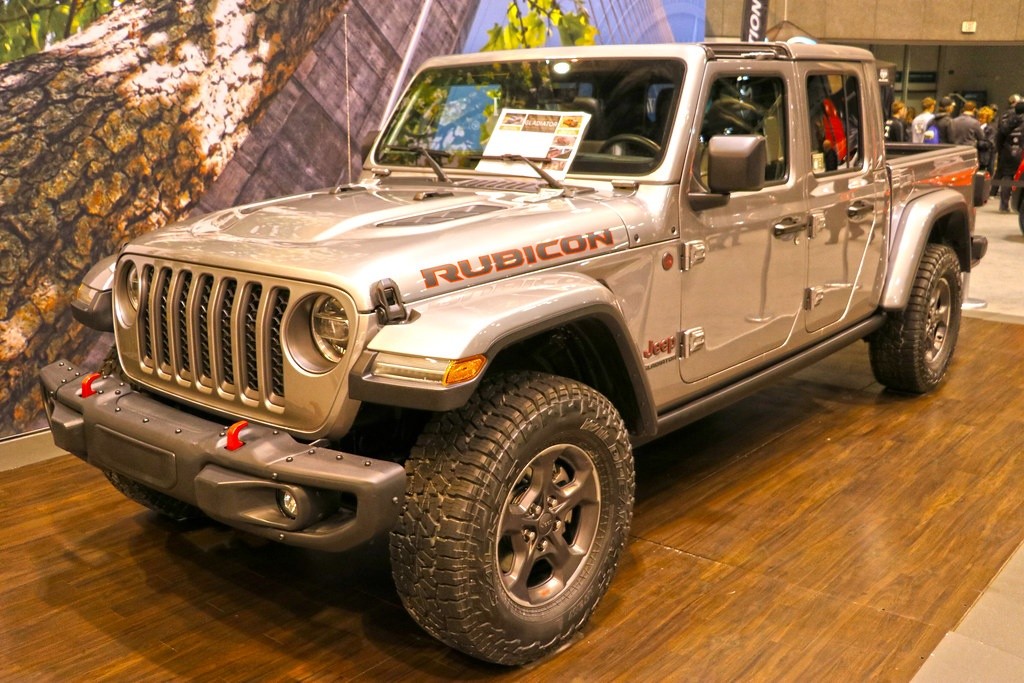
[36,38,992,673]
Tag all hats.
[940,95,954,107]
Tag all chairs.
[571,96,606,141]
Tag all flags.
[742,0,769,42]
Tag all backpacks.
[923,116,948,144]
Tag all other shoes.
[1000,206,1011,214]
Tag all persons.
[947,101,984,146]
[906,107,916,142]
[978,106,998,171]
[925,96,956,143]
[912,97,936,143]
[885,102,908,142]
[988,104,998,176]
[991,94,1024,211]
